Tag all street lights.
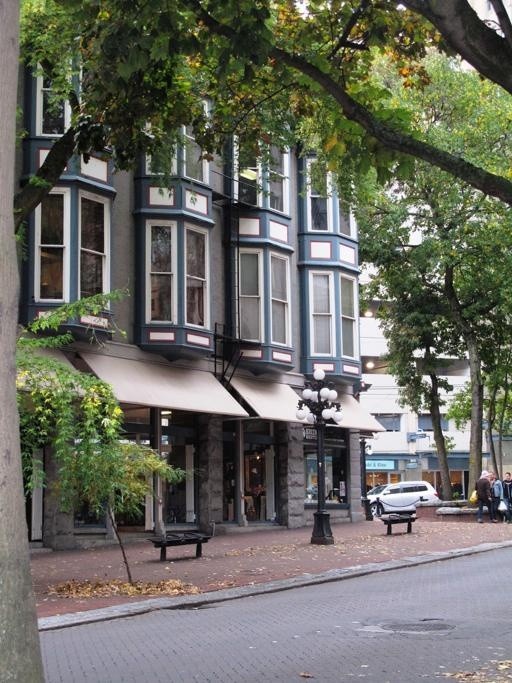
[360,433,380,497]
[295,370,343,511]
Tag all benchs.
[378,512,419,535]
[147,531,213,561]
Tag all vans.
[362,480,439,517]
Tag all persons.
[469,471,497,522]
[502,472,511,523]
[487,471,504,522]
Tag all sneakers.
[478,518,497,523]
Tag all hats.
[480,470,491,480]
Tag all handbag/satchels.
[498,500,508,511]
[469,490,478,503]
